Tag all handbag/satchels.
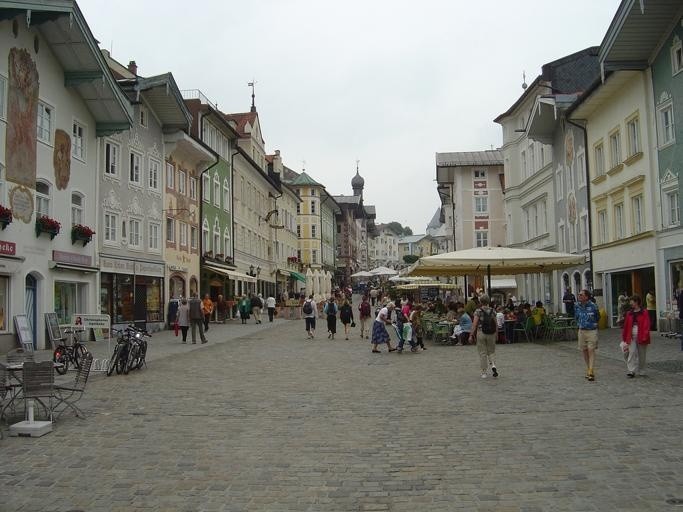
[619,341,629,363]
[402,322,413,341]
[397,311,410,323]
[174,319,179,337]
[351,321,357,327]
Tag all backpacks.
[327,302,334,315]
[477,308,497,334]
[303,301,314,314]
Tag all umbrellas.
[349,245,587,301]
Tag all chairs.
[0,348,93,423]
[419,310,579,345]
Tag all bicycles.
[49,325,90,375]
[103,322,152,376]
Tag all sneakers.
[480,366,498,379]
[307,331,371,340]
[371,346,427,353]
[584,371,648,382]
[182,340,208,345]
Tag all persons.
[323,286,354,340]
[621,295,652,378]
[562,285,577,326]
[616,289,629,328]
[574,289,602,381]
[238,292,276,324]
[175,293,213,344]
[357,287,545,353]
[299,294,318,338]
[646,287,657,331]
[468,294,499,378]
[217,294,227,323]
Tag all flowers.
[71,224,96,238]
[36,215,63,233]
[0,204,12,222]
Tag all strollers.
[390,324,418,352]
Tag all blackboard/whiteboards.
[44,312,62,341]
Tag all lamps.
[249,264,262,274]
